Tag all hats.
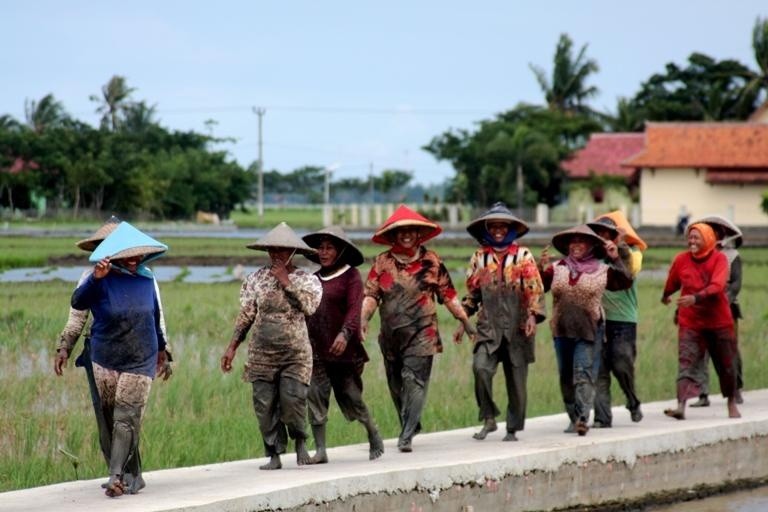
[247,222,319,255]
[552,224,607,257]
[586,209,647,251]
[466,202,530,245]
[77,215,167,262]
[685,216,742,259]
[371,204,442,247]
[301,225,365,266]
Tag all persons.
[690,224,744,406]
[358,226,476,453]
[307,244,384,461]
[70,255,167,496]
[660,224,741,419]
[452,220,547,442]
[54,256,171,491]
[533,236,634,435]
[220,248,323,468]
[591,231,643,428]
